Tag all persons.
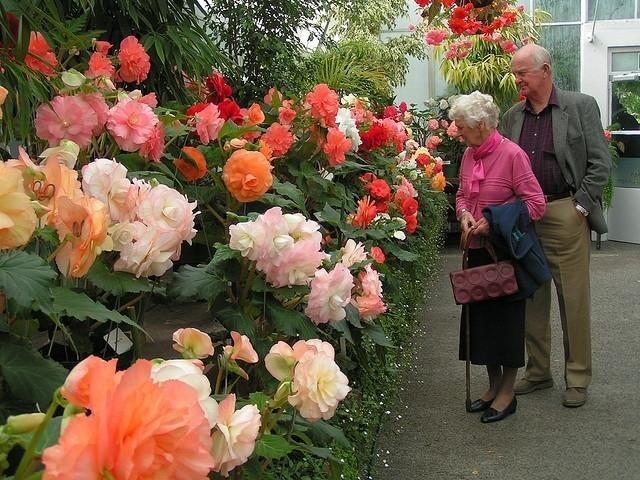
[501,43,614,407]
[447,89,547,423]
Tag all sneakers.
[562,385,589,408]
[511,377,555,395]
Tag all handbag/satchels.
[445,227,519,305]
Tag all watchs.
[576,204,589,218]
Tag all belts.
[542,190,575,204]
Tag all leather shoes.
[468,396,496,413]
[479,392,518,424]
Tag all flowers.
[604,125,625,211]
[0,0,446,480]
[440,0,552,155]
